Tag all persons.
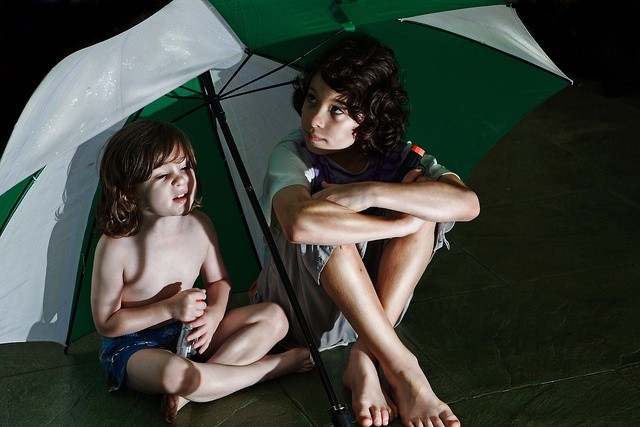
[248,30,481,426]
[90,118,316,422]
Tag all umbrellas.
[1,0,574,427]
